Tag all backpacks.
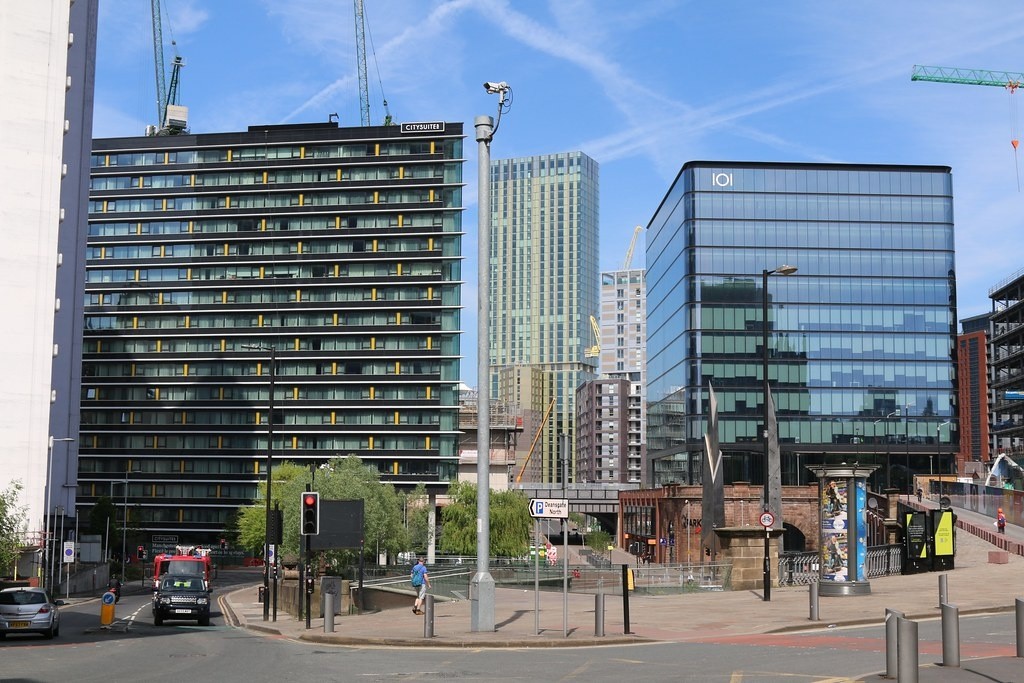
[411,565,423,587]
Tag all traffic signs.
[528,499,568,518]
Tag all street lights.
[104,481,128,562]
[44,436,75,588]
[905,400,916,503]
[762,264,799,601]
[241,342,275,620]
[887,411,897,489]
[937,421,951,509]
[120,470,143,586]
[874,419,883,493]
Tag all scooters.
[107,583,124,604]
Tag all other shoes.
[997,531,1004,534]
[412,607,417,614]
[416,610,424,615]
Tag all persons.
[108,574,121,602]
[572,570,580,579]
[827,536,844,569]
[998,508,1006,534]
[826,482,845,514]
[641,552,651,564]
[164,579,177,589]
[916,488,924,503]
[411,557,431,615]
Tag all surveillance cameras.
[483,82,508,94]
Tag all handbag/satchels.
[998,517,1004,525]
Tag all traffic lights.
[221,539,225,549]
[706,548,710,556]
[137,545,144,559]
[300,492,320,536]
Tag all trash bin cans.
[259,587,264,602]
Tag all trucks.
[150,544,216,587]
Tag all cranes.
[148,0,190,137]
[910,65,1024,95]
[353,0,398,126]
[623,225,645,269]
[584,315,601,359]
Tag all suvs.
[152,573,213,627]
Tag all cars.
[0,586,65,640]
